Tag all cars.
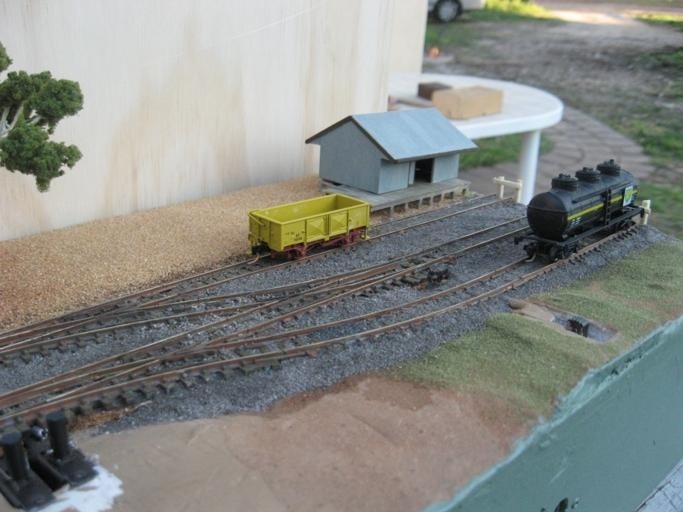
[427,0,485,22]
